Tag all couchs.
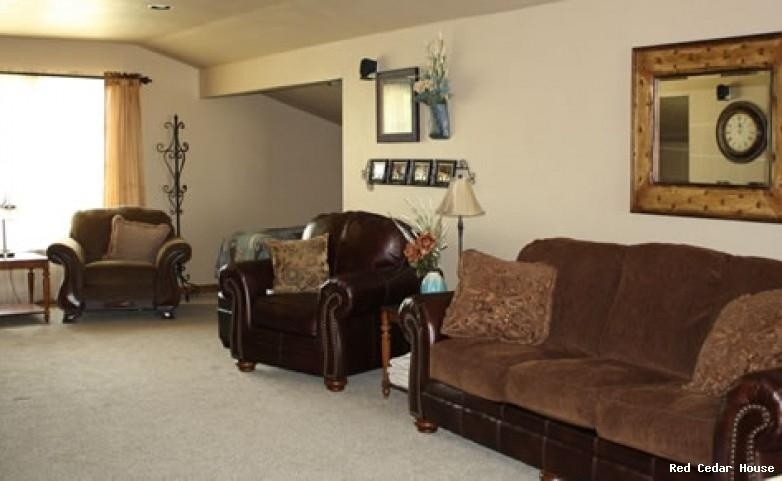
[399,238,782,481]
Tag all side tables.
[372,294,419,398]
[216,225,306,280]
[0,250,51,323]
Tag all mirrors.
[631,32,782,225]
[376,67,419,142]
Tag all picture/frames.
[362,159,457,189]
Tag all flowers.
[389,198,455,279]
[407,29,450,107]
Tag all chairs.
[217,210,430,391]
[47,207,192,324]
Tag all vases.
[419,270,447,296]
[429,104,449,139]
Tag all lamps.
[0,197,16,258]
[435,180,484,261]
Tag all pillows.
[263,233,330,295]
[682,287,781,399]
[439,249,558,346]
[101,215,172,264]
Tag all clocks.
[716,101,767,164]
